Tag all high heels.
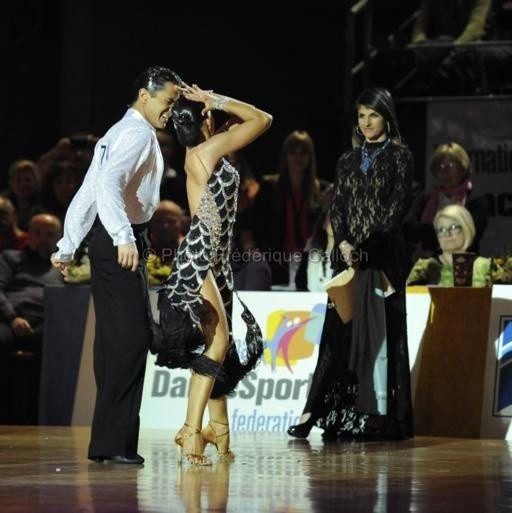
[199,419,235,459]
[174,423,213,468]
[284,409,332,440]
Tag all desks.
[40,282,512,441]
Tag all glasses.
[436,223,462,234]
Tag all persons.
[284,439,412,512]
[176,468,229,513]
[86,466,140,513]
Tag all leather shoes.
[86,453,146,465]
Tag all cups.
[451,250,474,285]
